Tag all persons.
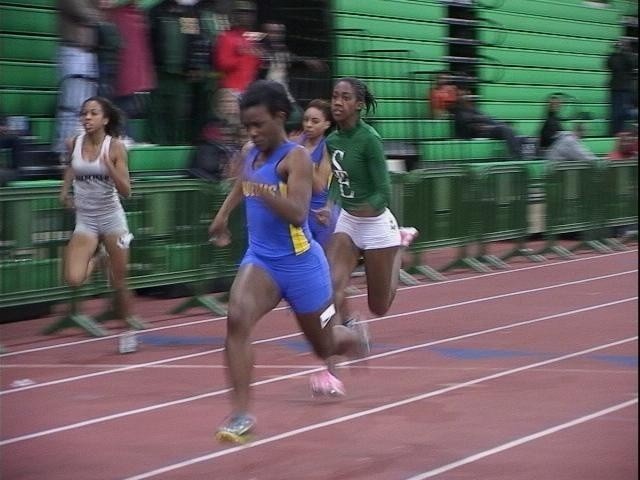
[0,116,64,186]
[604,30,639,158]
[537,96,597,160]
[430,71,523,160]
[51,0,327,264]
[57,94,141,355]
[291,99,350,323]
[207,77,370,446]
[306,78,419,401]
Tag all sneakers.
[400,227,420,246]
[216,416,256,444]
[310,371,345,397]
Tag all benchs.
[331,2,640,173]
[0,3,66,174]
[121,119,203,173]
